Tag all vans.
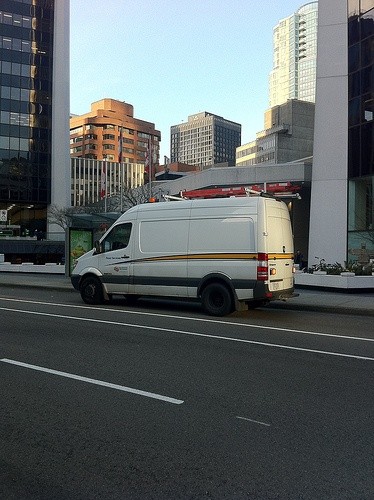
[71,196,297,315]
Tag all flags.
[101,125,149,200]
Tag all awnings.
[181,182,301,198]
